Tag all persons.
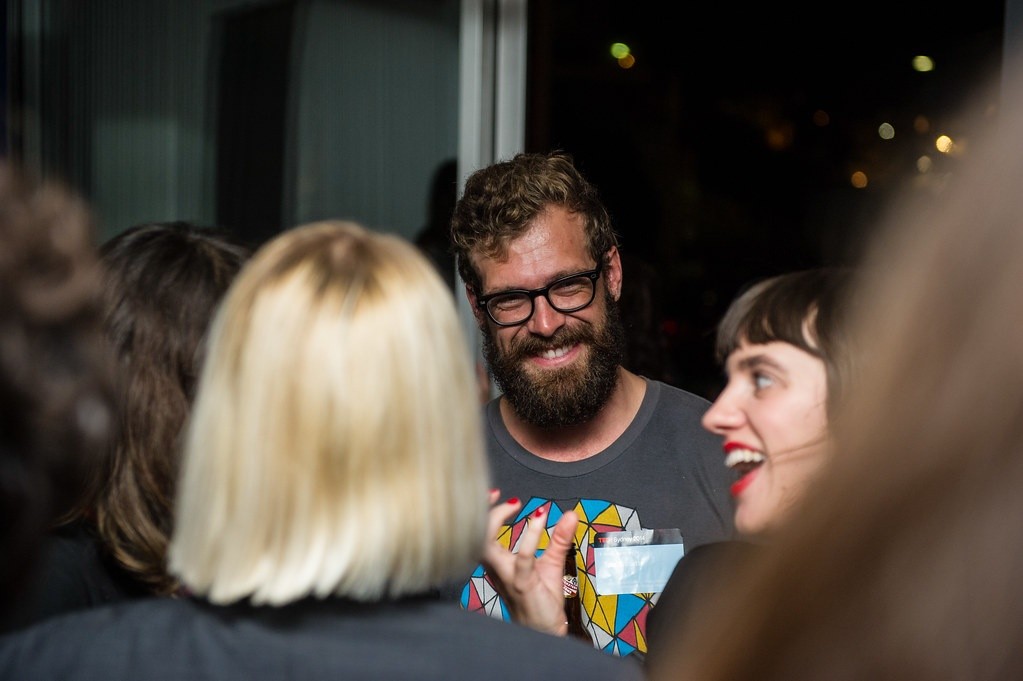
[87,221,248,597]
[461,147,736,670]
[0,218,645,679]
[0,161,142,641]
[652,59,1023,680]
[472,267,853,671]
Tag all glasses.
[474,257,603,327]
